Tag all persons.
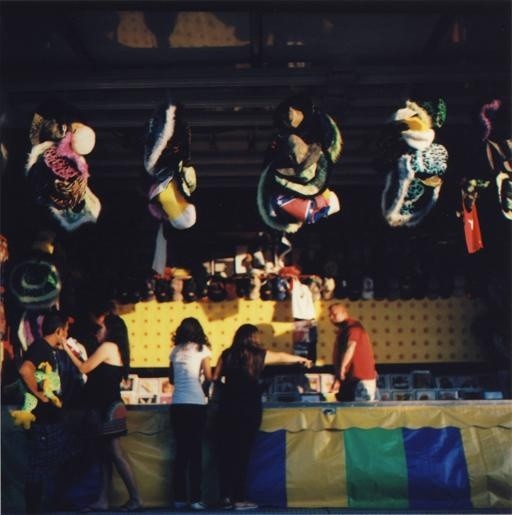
[1,93,512,513]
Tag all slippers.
[80,505,112,512]
[115,505,146,512]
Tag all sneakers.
[173,500,189,511]
[223,496,259,510]
[192,500,209,511]
